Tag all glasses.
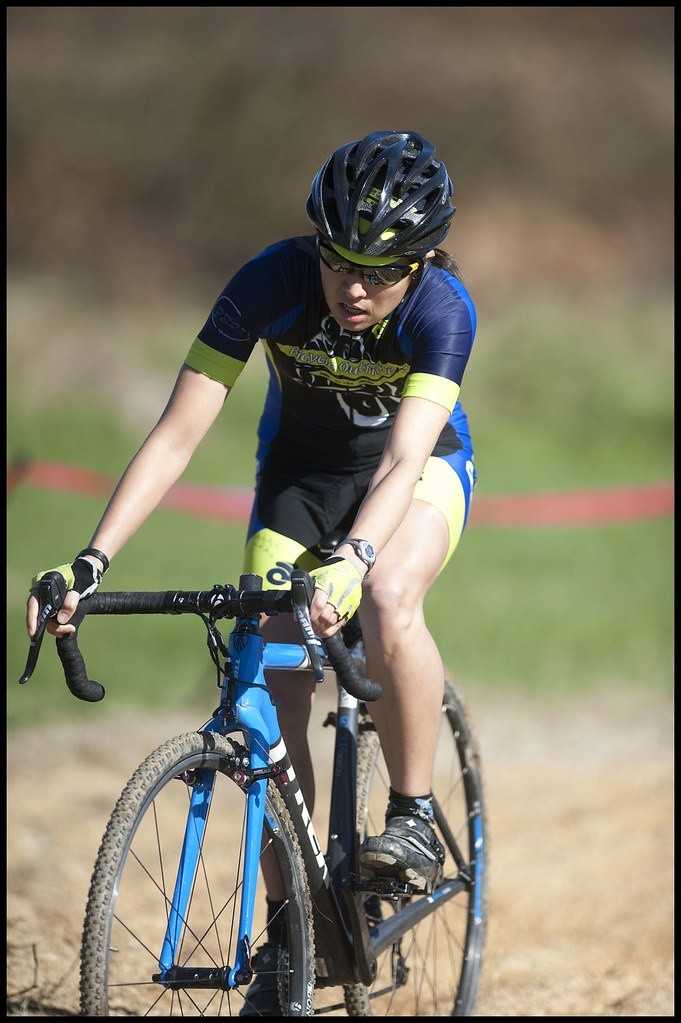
[316,235,426,289]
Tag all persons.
[27,130,477,1017]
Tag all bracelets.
[75,548,109,574]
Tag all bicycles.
[16,570,490,1016]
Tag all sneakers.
[239,943,291,1017]
[360,816,445,894]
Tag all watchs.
[331,538,376,576]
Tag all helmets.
[305,131,457,267]
